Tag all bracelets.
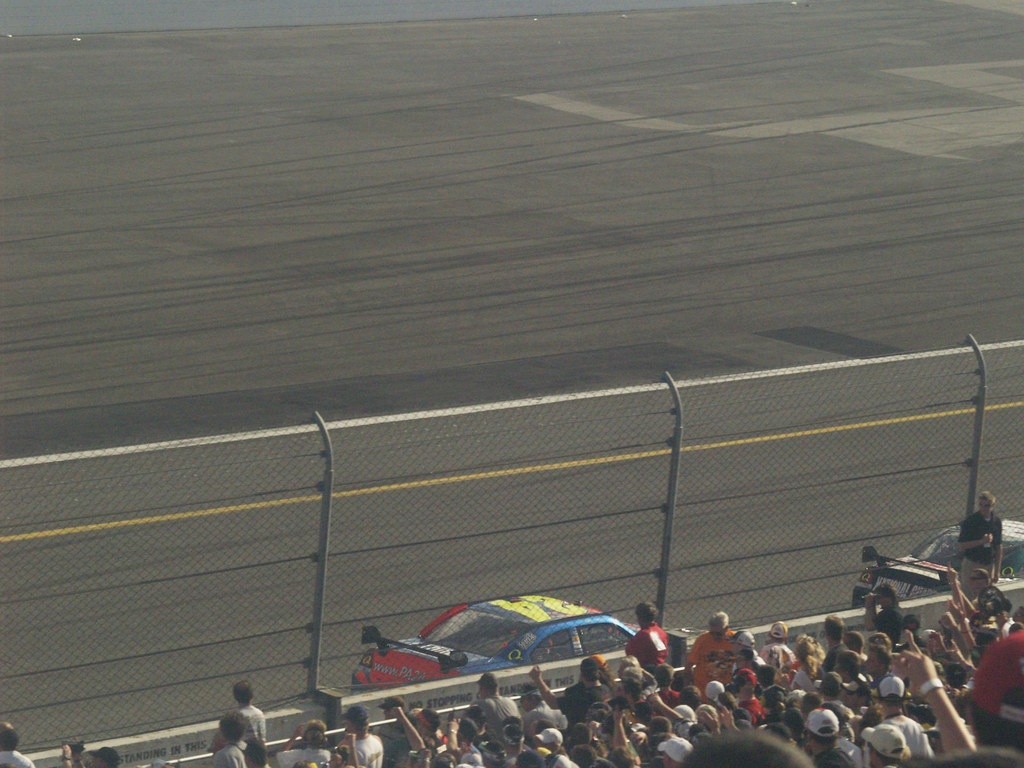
[448,729,457,736]
[919,678,943,694]
[948,581,957,584]
[74,759,81,762]
[64,756,71,760]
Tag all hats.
[910,702,937,727]
[919,630,936,639]
[705,680,725,701]
[734,668,757,684]
[880,676,905,697]
[377,696,405,707]
[786,689,806,701]
[535,728,563,746]
[673,705,698,722]
[728,631,756,648]
[647,716,671,732]
[340,705,370,723]
[771,621,788,638]
[709,612,729,630]
[630,723,646,732]
[629,601,658,618]
[476,672,497,687]
[861,724,906,757]
[843,681,858,691]
[657,737,694,762]
[804,709,839,736]
[512,682,540,697]
[88,747,120,766]
[591,654,608,669]
[974,630,1024,722]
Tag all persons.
[958,491,1003,599]
[625,601,670,670]
[684,612,737,704]
[62,744,120,768]
[276,562,1024,768]
[211,711,251,768]
[864,583,903,647]
[232,680,266,742]
[0,722,36,768]
[245,737,270,768]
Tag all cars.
[852,520,1024,610]
[351,594,642,696]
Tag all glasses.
[1014,613,1024,617]
[979,503,991,507]
[969,577,985,581]
